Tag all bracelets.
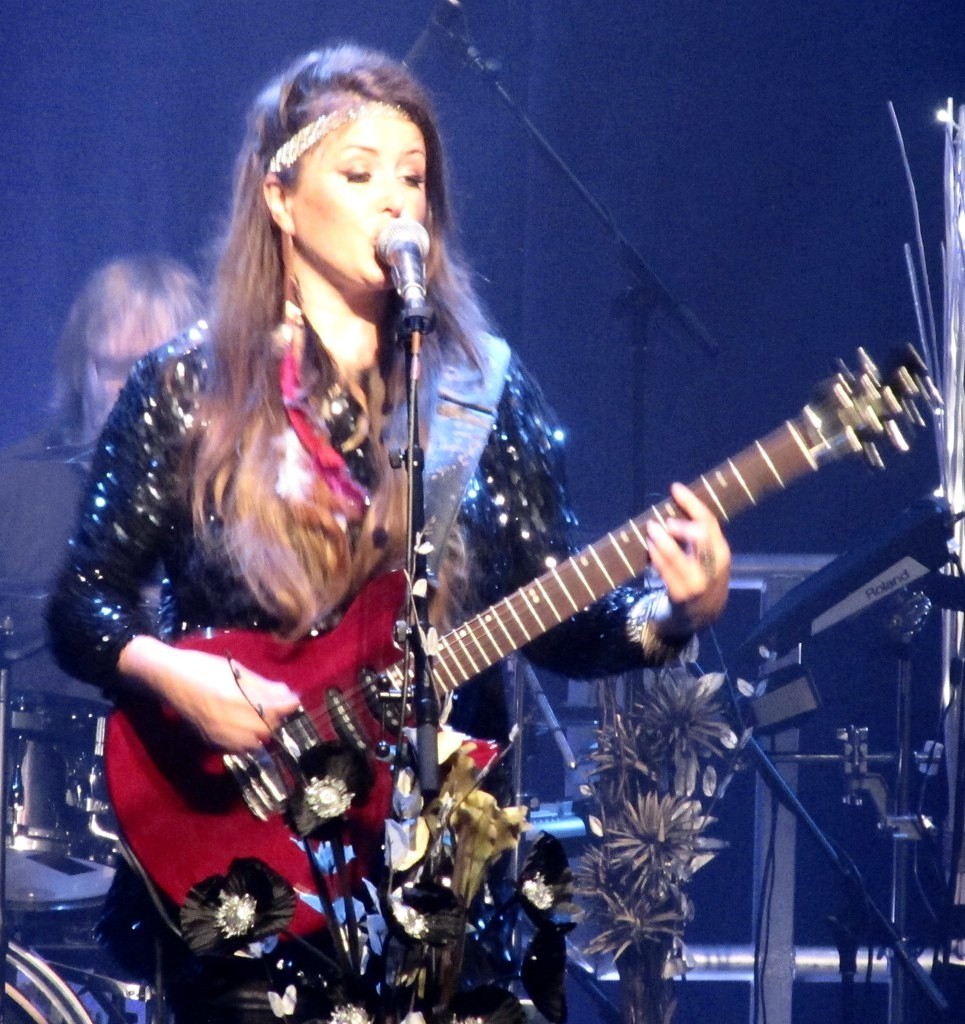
[627,591,692,665]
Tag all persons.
[1,254,214,692]
[44,44,731,1024]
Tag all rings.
[697,551,714,574]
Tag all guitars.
[102,343,946,954]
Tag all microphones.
[401,0,460,74]
[374,217,431,331]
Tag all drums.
[2,677,122,915]
